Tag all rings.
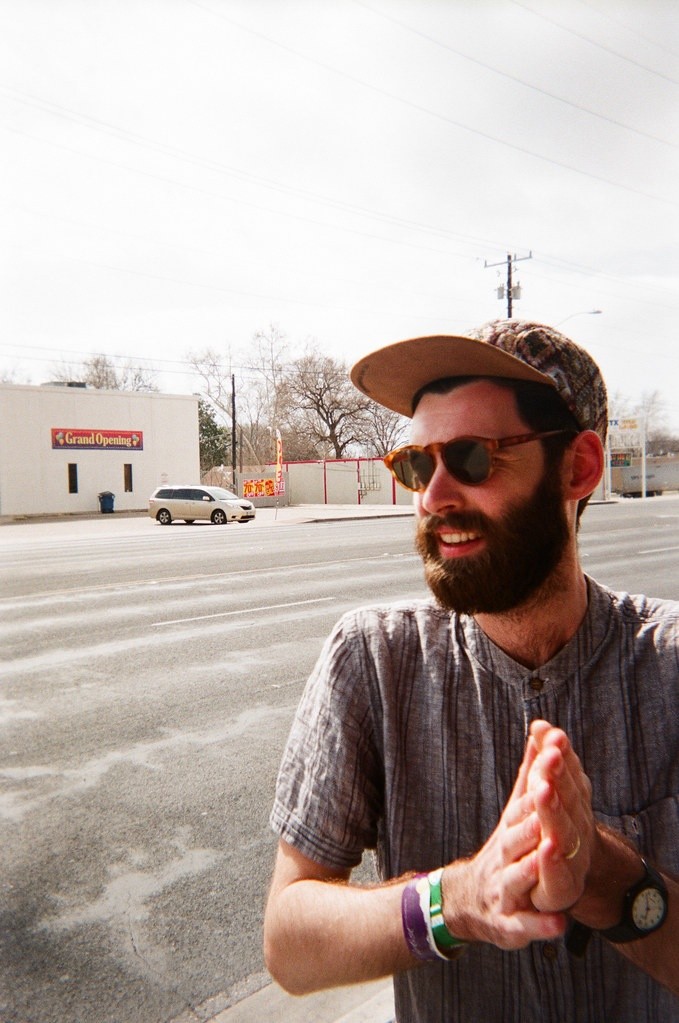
[564,827,582,860]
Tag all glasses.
[384,428,580,493]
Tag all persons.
[259,319,679,1023]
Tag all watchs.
[591,852,669,943]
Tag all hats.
[349,317,608,450]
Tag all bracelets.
[402,866,468,965]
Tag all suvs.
[148,485,255,526]
